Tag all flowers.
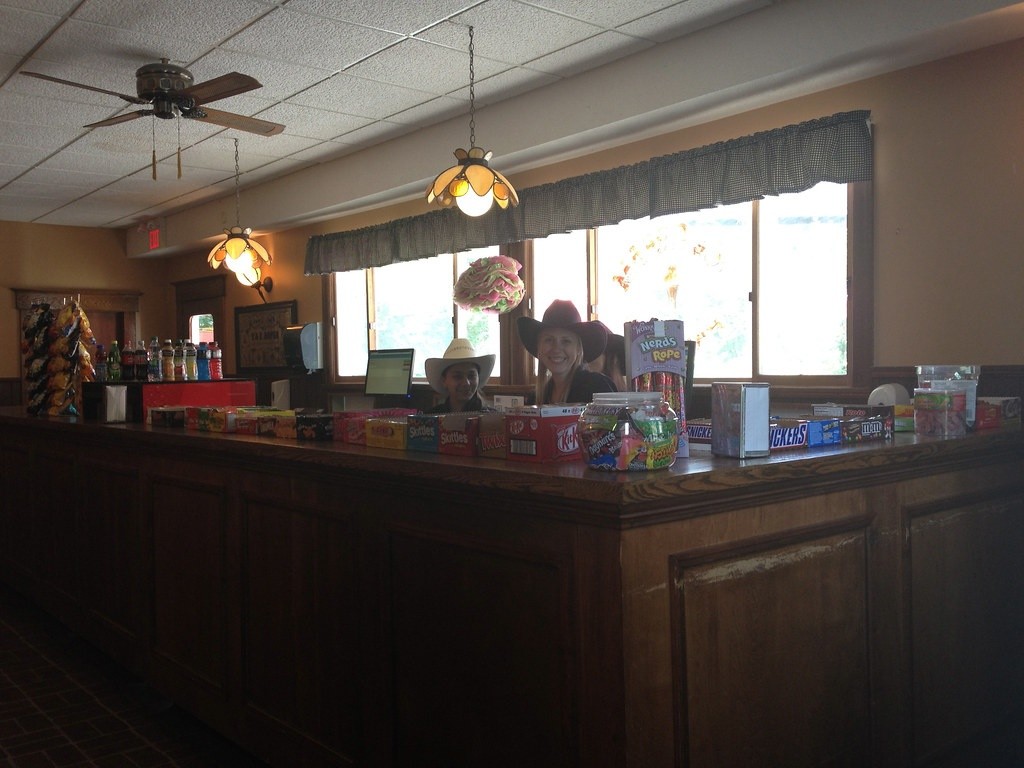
[452,254,526,315]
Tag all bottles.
[174,339,188,380]
[147,337,163,381]
[121,340,134,381]
[196,342,211,380]
[575,391,678,469]
[184,339,198,380]
[134,341,147,380]
[107,341,121,381]
[94,345,107,380]
[209,342,223,380]
[162,339,175,380]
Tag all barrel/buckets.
[913,388,967,435]
[915,364,981,431]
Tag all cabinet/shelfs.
[0,401,1024,768]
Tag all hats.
[425,338,496,396]
[517,299,607,363]
[591,319,626,376]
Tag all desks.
[80,375,256,427]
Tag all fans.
[18,59,277,137]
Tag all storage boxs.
[147,320,1022,473]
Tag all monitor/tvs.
[363,348,415,396]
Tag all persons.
[518,299,618,402]
[425,338,496,413]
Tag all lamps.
[424,26,520,219]
[234,267,273,295]
[206,140,270,272]
